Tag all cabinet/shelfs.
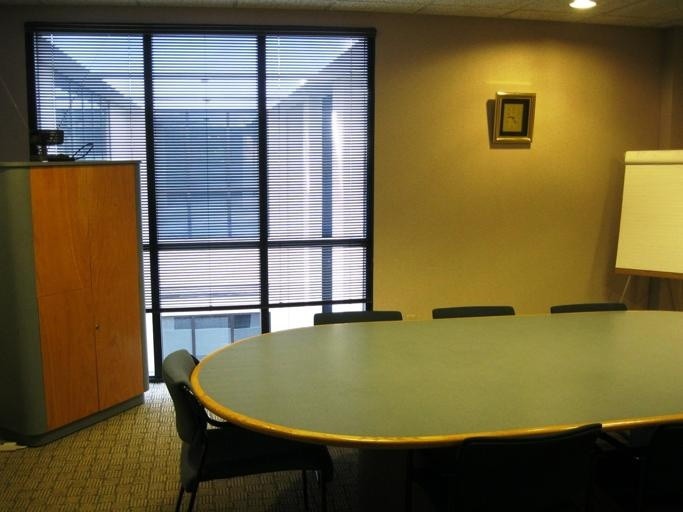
[0,161,149,447]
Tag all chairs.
[163,303,683,512]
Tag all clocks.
[493,92,536,144]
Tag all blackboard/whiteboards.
[614,148,683,280]
